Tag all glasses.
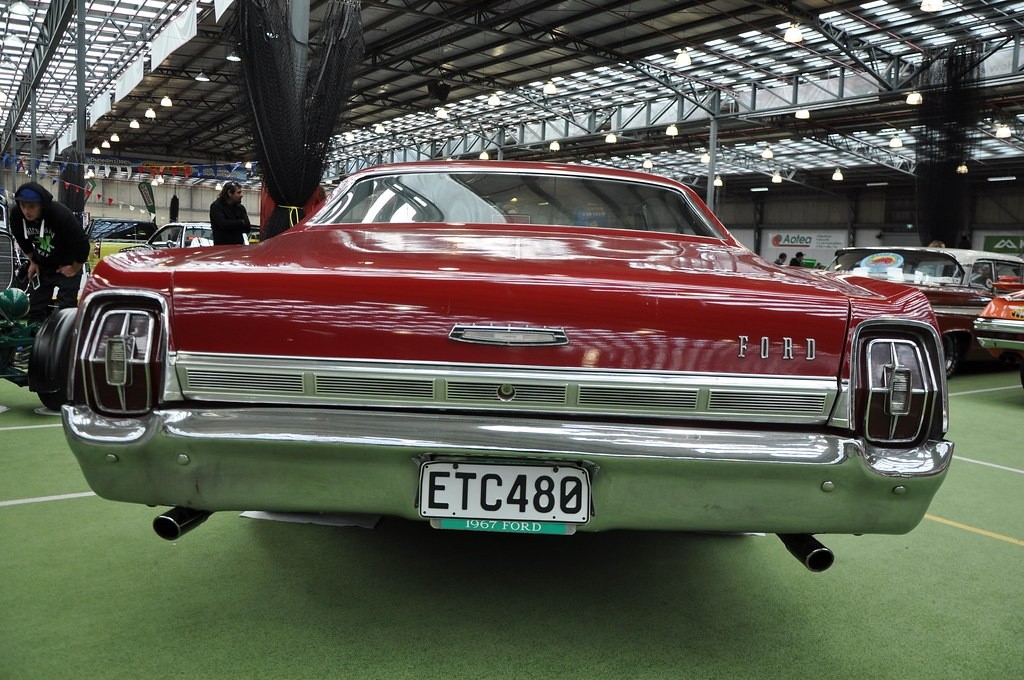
[231,182,238,187]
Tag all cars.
[148,223,262,285]
[65,161,954,575]
[835,245,1020,374]
[89,217,159,281]
[973,293,1018,364]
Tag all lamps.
[226,47,242,62]
[476,0,1012,188]
[91,95,175,155]
[194,69,211,82]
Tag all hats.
[796,252,805,257]
[13,188,42,202]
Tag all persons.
[815,263,821,268]
[789,252,805,267]
[914,241,953,278]
[774,253,786,265]
[9,181,91,364]
[210,182,251,246]
